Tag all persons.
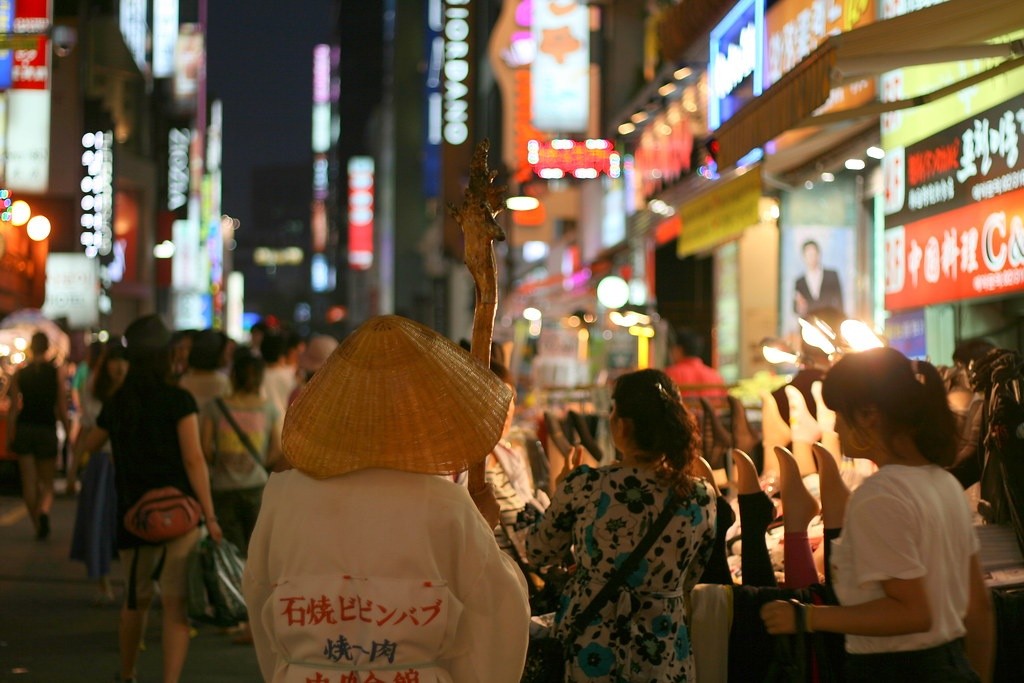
[203,350,286,631]
[9,330,71,537]
[243,314,532,683]
[532,380,854,588]
[173,328,549,553]
[795,243,843,319]
[759,342,993,683]
[943,338,995,489]
[527,368,719,683]
[84,312,223,682]
[74,344,125,603]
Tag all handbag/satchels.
[186,536,250,626]
[521,636,568,682]
[122,486,202,543]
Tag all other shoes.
[36,512,49,539]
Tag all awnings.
[709,0,1024,177]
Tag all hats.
[279,315,514,481]
[120,314,181,362]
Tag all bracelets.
[207,517,218,521]
[806,605,816,633]
